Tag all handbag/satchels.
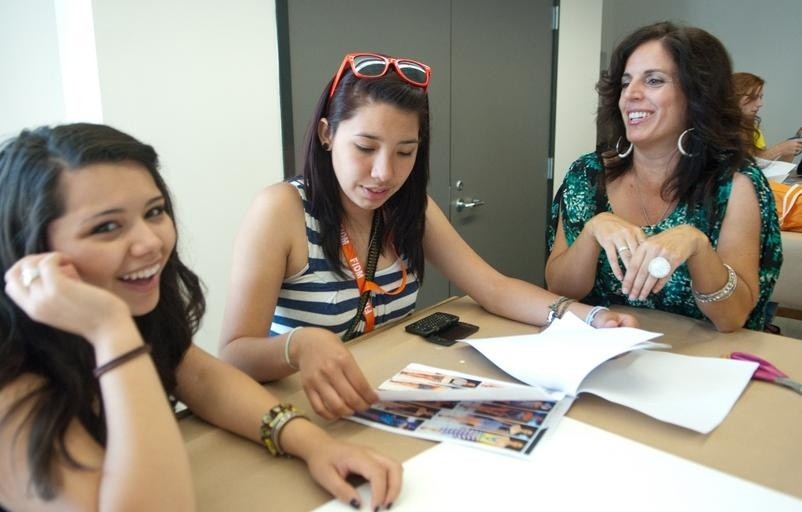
[767,181,802,232]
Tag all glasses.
[327,52,432,102]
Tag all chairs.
[764,227,802,340]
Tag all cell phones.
[405,312,479,346]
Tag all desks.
[172,287,802,512]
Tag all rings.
[617,246,630,253]
[21,267,43,289]
[649,258,671,279]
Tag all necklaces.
[629,160,678,230]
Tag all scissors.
[731,352,802,395]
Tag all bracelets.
[91,342,153,379]
[259,403,312,461]
[557,299,579,320]
[283,326,305,370]
[544,294,578,328]
[585,302,612,327]
[688,263,738,304]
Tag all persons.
[0,121,405,511]
[544,19,784,335]
[216,54,639,427]
[730,73,802,163]
